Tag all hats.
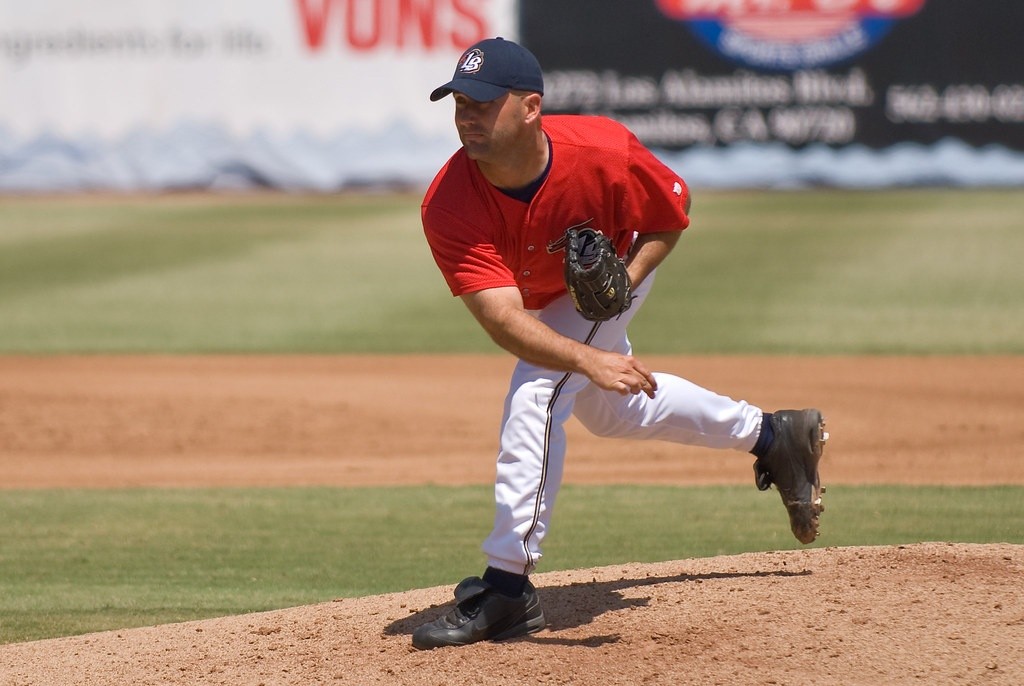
[430,37,544,102]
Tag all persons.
[411,36,828,651]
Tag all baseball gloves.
[563,228,638,322]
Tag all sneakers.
[753,408,829,544]
[411,576,545,652]
